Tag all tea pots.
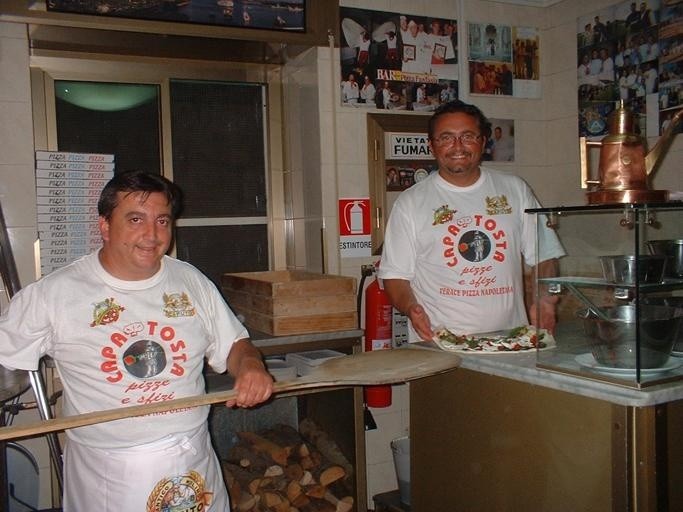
[578,97,682,204]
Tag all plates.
[573,352,681,375]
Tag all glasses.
[431,130,482,143]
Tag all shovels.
[0,348,461,441]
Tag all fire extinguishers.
[357,260,392,407]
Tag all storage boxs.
[220,267,358,338]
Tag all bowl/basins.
[643,239,682,277]
[598,254,669,283]
[576,305,681,368]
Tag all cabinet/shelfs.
[188,351,366,511]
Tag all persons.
[379,101,569,344]
[467,22,542,96]
[339,74,360,105]
[383,82,394,109]
[415,83,428,105]
[385,167,399,186]
[356,30,372,73]
[399,15,459,65]
[440,82,449,102]
[360,74,377,109]
[382,28,401,71]
[448,83,458,102]
[575,0,683,135]
[483,125,509,162]
[0,169,273,512]
